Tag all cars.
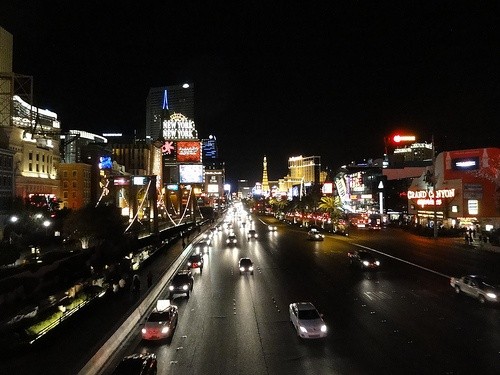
[168,270,195,296]
[111,353,159,375]
[268,224,277,231]
[247,230,259,240]
[140,300,179,342]
[308,229,325,241]
[288,302,328,339]
[348,249,381,269]
[238,257,255,275]
[449,274,500,307]
[225,233,238,246]
[187,252,204,271]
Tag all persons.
[439,225,488,249]
[118,277,126,295]
[147,271,153,289]
[181,233,185,244]
[133,276,140,298]
[113,281,119,299]
[132,272,141,286]
[126,275,133,296]
[187,230,190,239]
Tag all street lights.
[160,82,190,141]
[34,213,43,263]
[9,216,19,274]
[43,220,51,249]
